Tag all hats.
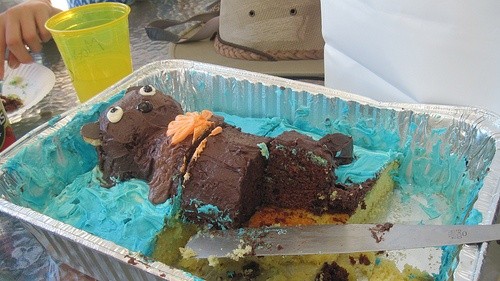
[170,1,327,78]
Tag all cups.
[44,3,134,105]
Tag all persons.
[0,0,138,80]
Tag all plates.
[3,59,56,119]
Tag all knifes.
[184,221,500,252]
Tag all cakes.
[32,79,400,281]
